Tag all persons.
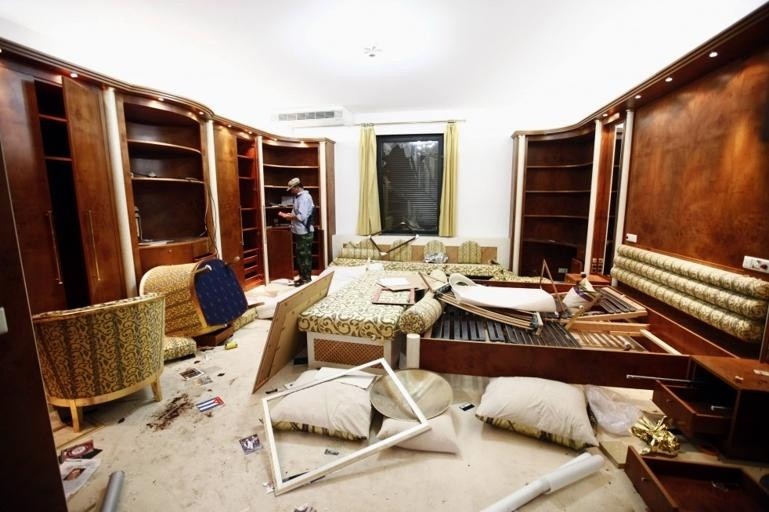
[243,439,254,453]
[250,436,261,448]
[278,177,317,288]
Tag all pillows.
[256,366,383,444]
[163,335,197,361]
[399,297,443,335]
[562,277,603,317]
[374,406,462,456]
[474,376,600,452]
[333,234,482,264]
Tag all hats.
[286,177,301,192]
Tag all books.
[177,366,205,383]
[195,396,225,416]
[58,439,95,466]
[598,438,631,470]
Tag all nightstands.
[565,271,610,287]
[652,354,769,466]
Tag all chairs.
[31,292,167,433]
[138,248,266,338]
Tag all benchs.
[297,274,415,375]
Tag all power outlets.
[741,254,769,274]
[625,233,637,243]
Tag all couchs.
[328,244,505,280]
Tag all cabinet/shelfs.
[215,119,268,292]
[1,41,121,316]
[604,128,623,275]
[102,76,219,295]
[508,118,596,280]
[262,125,336,280]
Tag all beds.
[406,241,768,388]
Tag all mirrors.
[596,117,626,280]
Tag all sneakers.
[294,278,308,287]
